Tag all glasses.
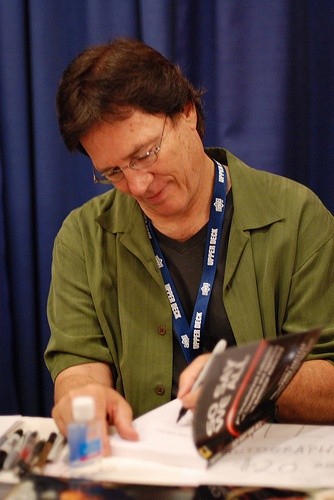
[91,115,170,185]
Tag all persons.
[45,39,334,456]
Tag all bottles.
[68,397,106,499]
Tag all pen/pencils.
[175,339,228,421]
[0,429,67,480]
